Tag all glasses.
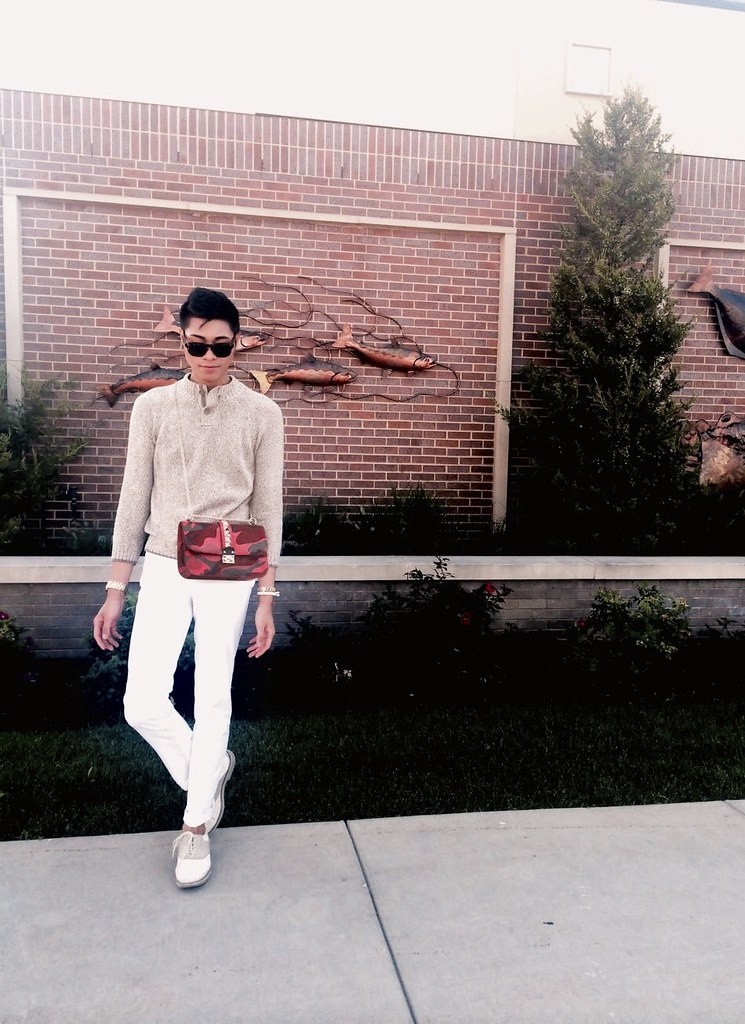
[183,333,239,358]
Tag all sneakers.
[173,748,235,889]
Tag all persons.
[92,288,283,889]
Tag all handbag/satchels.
[174,520,270,580]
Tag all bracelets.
[106,581,128,593]
[257,586,281,597]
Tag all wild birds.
[94,362,191,408]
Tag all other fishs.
[331,321,438,375]
[249,352,358,394]
[153,304,268,353]
[686,257,745,362]
